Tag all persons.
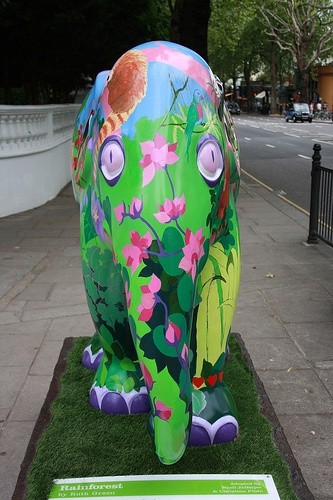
[323,101,328,111]
[316,102,321,111]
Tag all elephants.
[70,40,240,466]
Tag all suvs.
[284,102,314,123]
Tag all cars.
[228,103,240,115]
